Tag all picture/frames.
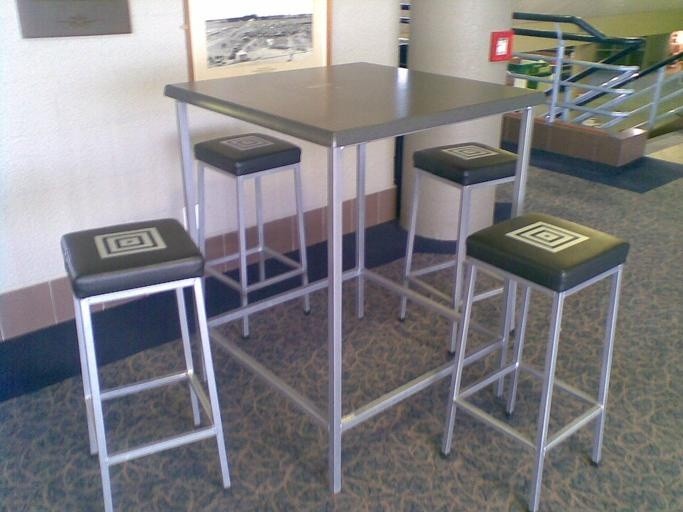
[182,0,334,82]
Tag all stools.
[193,133,311,339]
[440,211,629,512]
[398,141,518,355]
[61,218,232,511]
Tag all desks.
[164,62,546,495]
